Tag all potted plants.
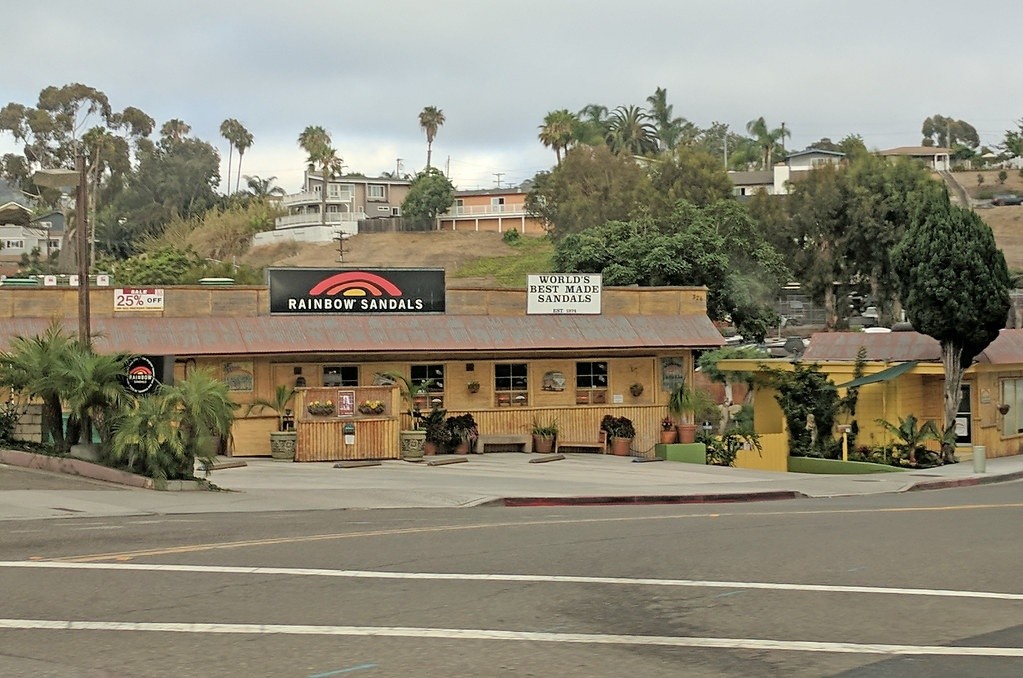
[630,383,643,396]
[601,414,636,456]
[382,369,435,461]
[344,424,355,445]
[668,382,721,444]
[521,415,564,453]
[244,384,297,459]
[407,405,447,456]
[154,361,242,457]
[446,413,479,454]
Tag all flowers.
[661,414,674,431]
[467,380,480,390]
[358,399,384,411]
[307,399,335,409]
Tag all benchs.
[551,431,607,454]
[471,434,533,454]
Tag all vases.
[470,388,478,393]
[360,407,383,414]
[661,429,677,444]
[308,407,333,416]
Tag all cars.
[992,194,1023,206]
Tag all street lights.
[34,157,90,355]
